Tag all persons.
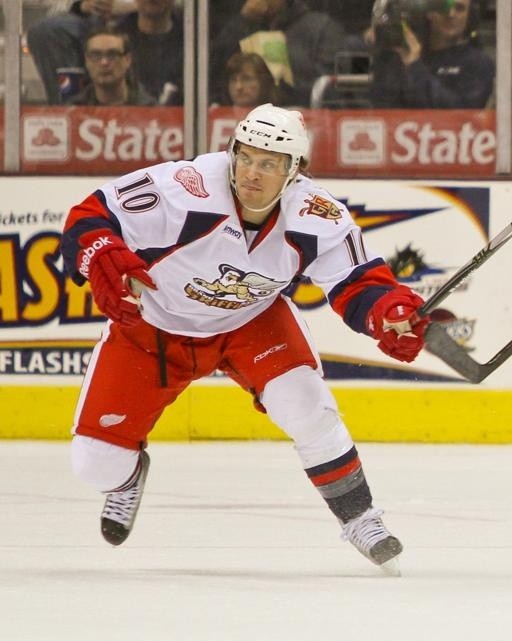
[27,1,349,109]
[345,1,494,108]
[60,103,432,566]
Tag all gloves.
[75,227,158,328]
[365,285,429,363]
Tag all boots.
[339,504,403,566]
[101,451,150,546]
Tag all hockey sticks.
[425,323,511,385]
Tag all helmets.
[235,103,309,156]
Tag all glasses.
[87,48,127,59]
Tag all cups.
[55,67,86,104]
